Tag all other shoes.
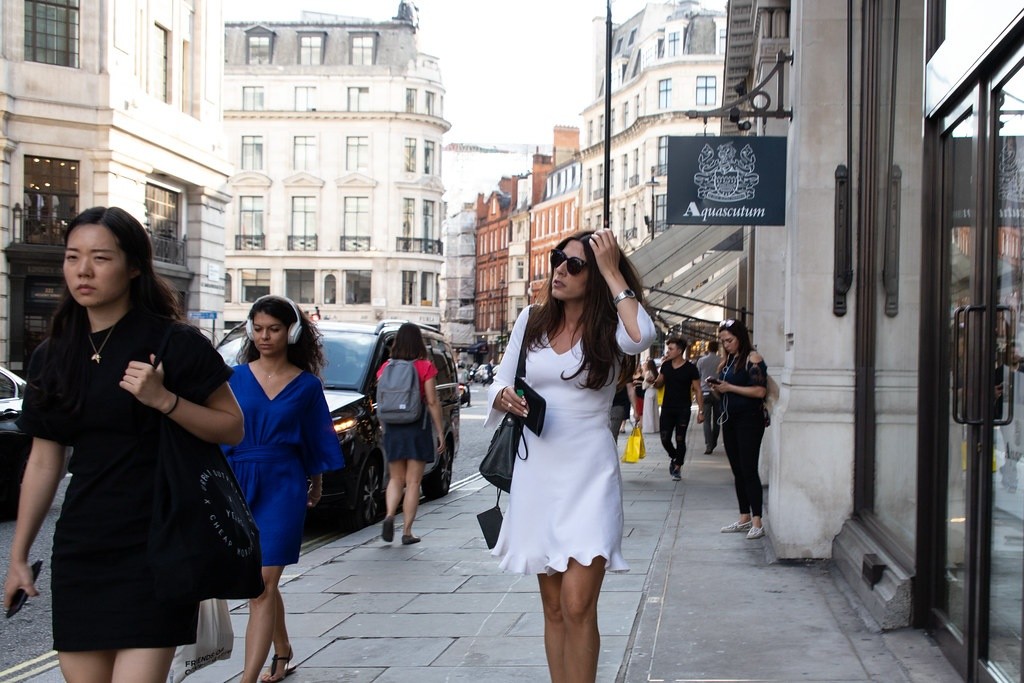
[402,534,421,544]
[746,526,764,538]
[720,521,752,532]
[670,457,681,480]
[382,517,394,541]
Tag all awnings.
[625,224,743,291]
[655,250,747,335]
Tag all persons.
[6,207,245,682]
[483,229,658,683]
[705,318,768,539]
[483,358,496,387]
[609,358,660,445]
[647,336,705,481]
[218,297,346,683]
[696,341,725,454]
[375,323,447,545]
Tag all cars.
[472,364,496,382]
[0,365,73,521]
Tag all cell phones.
[6,560,43,619]
[707,378,721,385]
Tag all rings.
[507,402,512,408]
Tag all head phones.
[244,293,303,344]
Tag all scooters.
[457,371,474,406]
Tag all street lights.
[499,278,506,363]
[644,166,660,322]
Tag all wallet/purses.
[512,374,547,438]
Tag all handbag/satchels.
[477,506,503,549]
[150,422,266,609]
[171,598,235,682]
[479,412,523,493]
[622,416,646,463]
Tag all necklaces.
[87,324,116,364]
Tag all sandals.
[260,645,296,682]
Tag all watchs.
[613,289,636,306]
[698,411,704,414]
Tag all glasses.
[550,249,588,275]
[719,320,737,327]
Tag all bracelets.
[161,394,178,415]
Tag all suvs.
[212,309,461,533]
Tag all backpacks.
[745,350,780,427]
[376,359,422,424]
